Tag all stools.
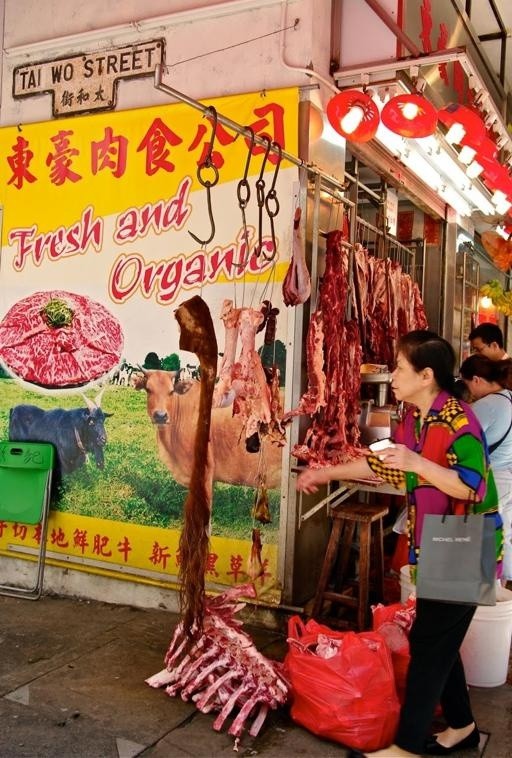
[309,503,389,634]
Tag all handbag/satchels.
[415,489,496,607]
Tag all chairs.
[0,439,56,601]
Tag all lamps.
[323,83,512,243]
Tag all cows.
[132,362,287,534]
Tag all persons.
[294,328,501,757]
[455,352,511,590]
[468,320,511,389]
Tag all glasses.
[472,343,490,354]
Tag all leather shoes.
[427,720,480,755]
[346,749,367,758]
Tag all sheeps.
[7,389,113,486]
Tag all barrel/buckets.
[461,584,512,688]
[399,565,416,602]
